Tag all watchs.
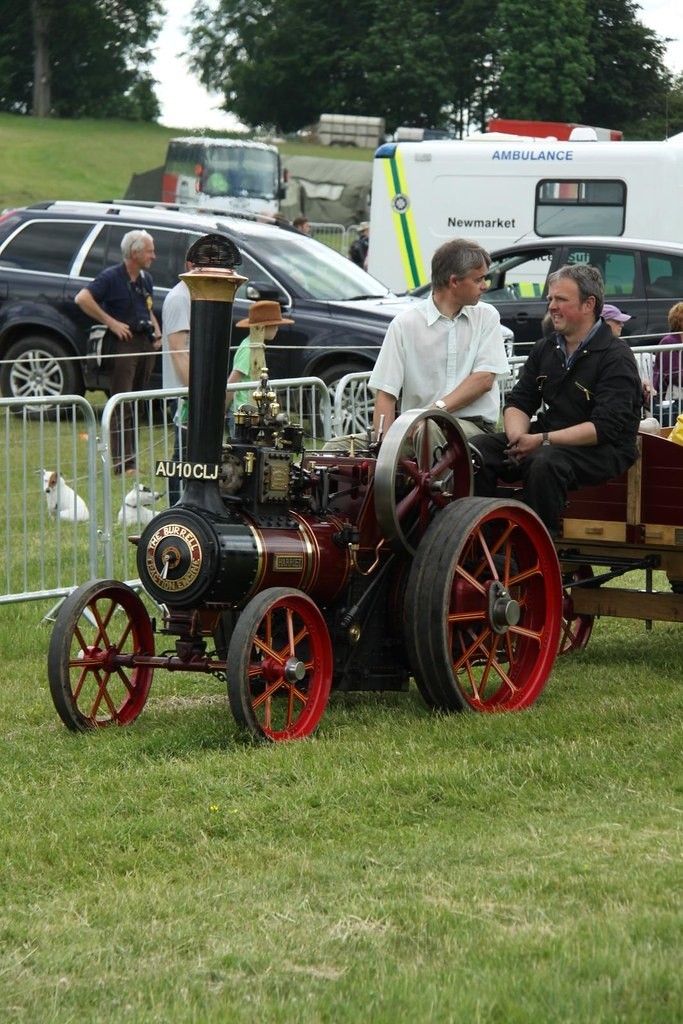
[433,400,448,412]
[541,432,551,446]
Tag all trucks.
[367,120,683,298]
[163,137,290,225]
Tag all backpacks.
[351,235,368,265]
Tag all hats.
[356,222,369,232]
[235,300,296,328]
[599,304,632,323]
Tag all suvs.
[0,200,514,434]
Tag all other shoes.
[479,550,524,580]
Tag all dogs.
[33,469,89,523]
[117,482,166,527]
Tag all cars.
[403,238,682,348]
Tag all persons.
[350,221,368,270]
[226,301,294,438]
[322,239,507,510]
[274,214,290,226]
[162,234,244,510]
[293,218,311,235]
[541,302,683,399]
[464,264,643,587]
[74,230,163,476]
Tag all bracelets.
[157,336,161,341]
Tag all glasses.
[610,320,620,325]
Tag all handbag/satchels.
[86,324,120,375]
